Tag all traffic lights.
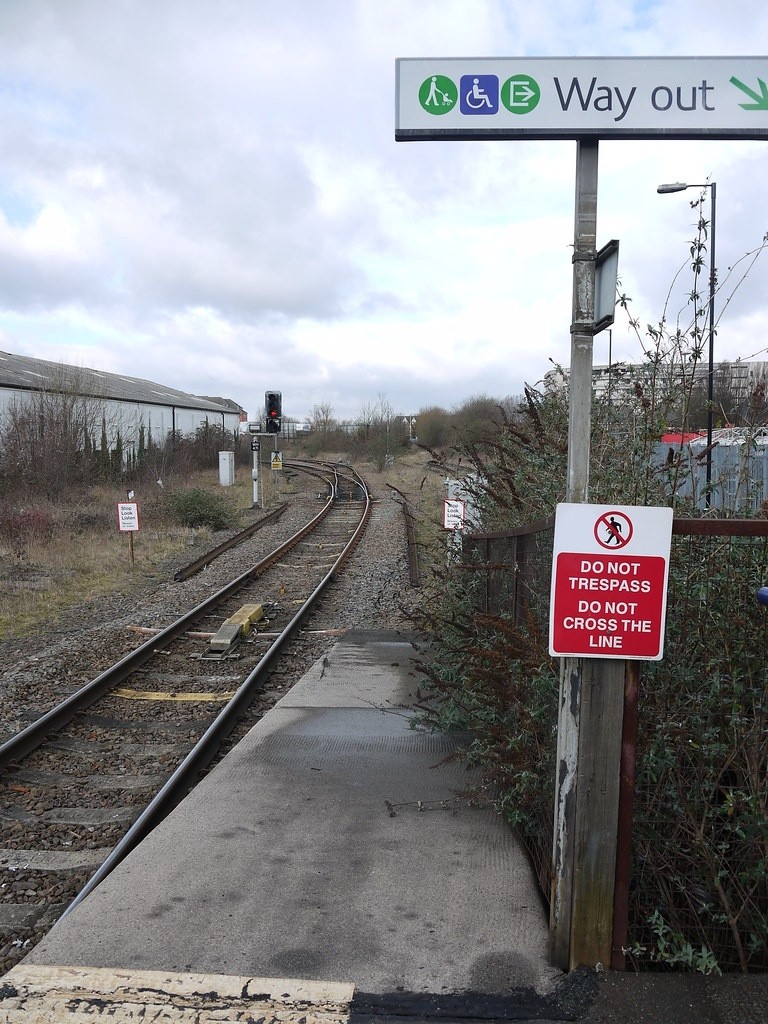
[264,390,282,433]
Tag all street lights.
[657,181,718,519]
